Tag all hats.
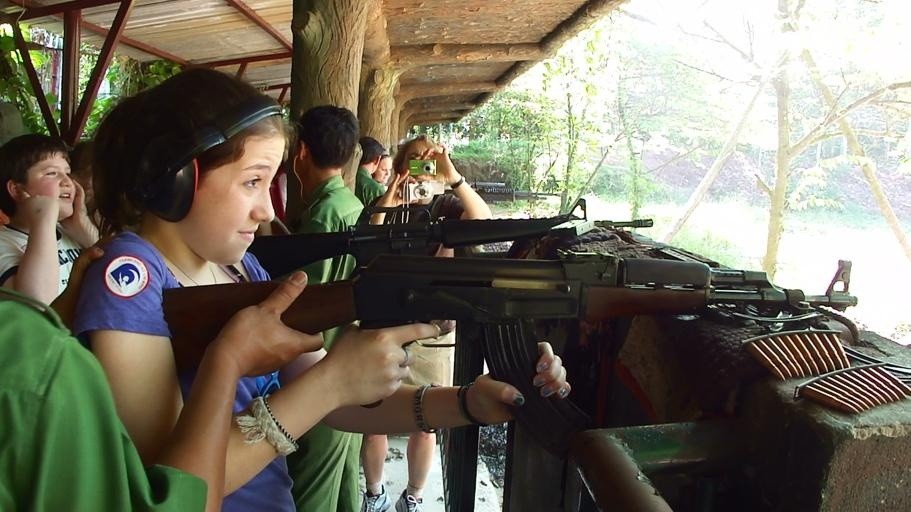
[360,137,389,162]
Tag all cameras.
[408,159,437,176]
[409,181,445,203]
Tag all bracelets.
[454,380,489,432]
[412,380,444,436]
[231,394,303,459]
[449,175,467,190]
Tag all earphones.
[295,152,300,158]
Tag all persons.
[268,156,292,236]
[67,64,572,510]
[342,130,491,512]
[252,100,375,511]
[1,278,327,510]
[354,134,387,207]
[64,142,112,241]
[0,132,103,315]
[370,147,392,193]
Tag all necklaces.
[156,253,220,287]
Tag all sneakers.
[395,491,424,512]
[360,485,391,511]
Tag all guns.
[247,199,653,281]
[161,248,858,461]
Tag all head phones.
[129,95,286,224]
